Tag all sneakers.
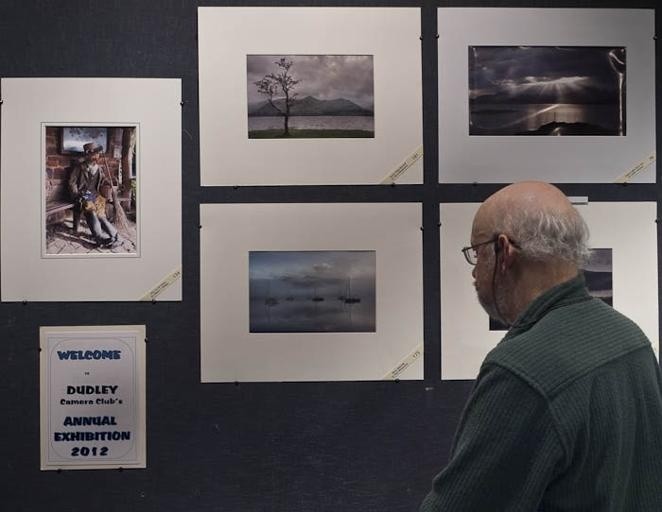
[97,234,121,248]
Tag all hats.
[82,143,103,156]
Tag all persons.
[417,178,662,512]
[66,143,120,248]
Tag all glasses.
[461,239,522,265]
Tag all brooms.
[99,143,137,255]
